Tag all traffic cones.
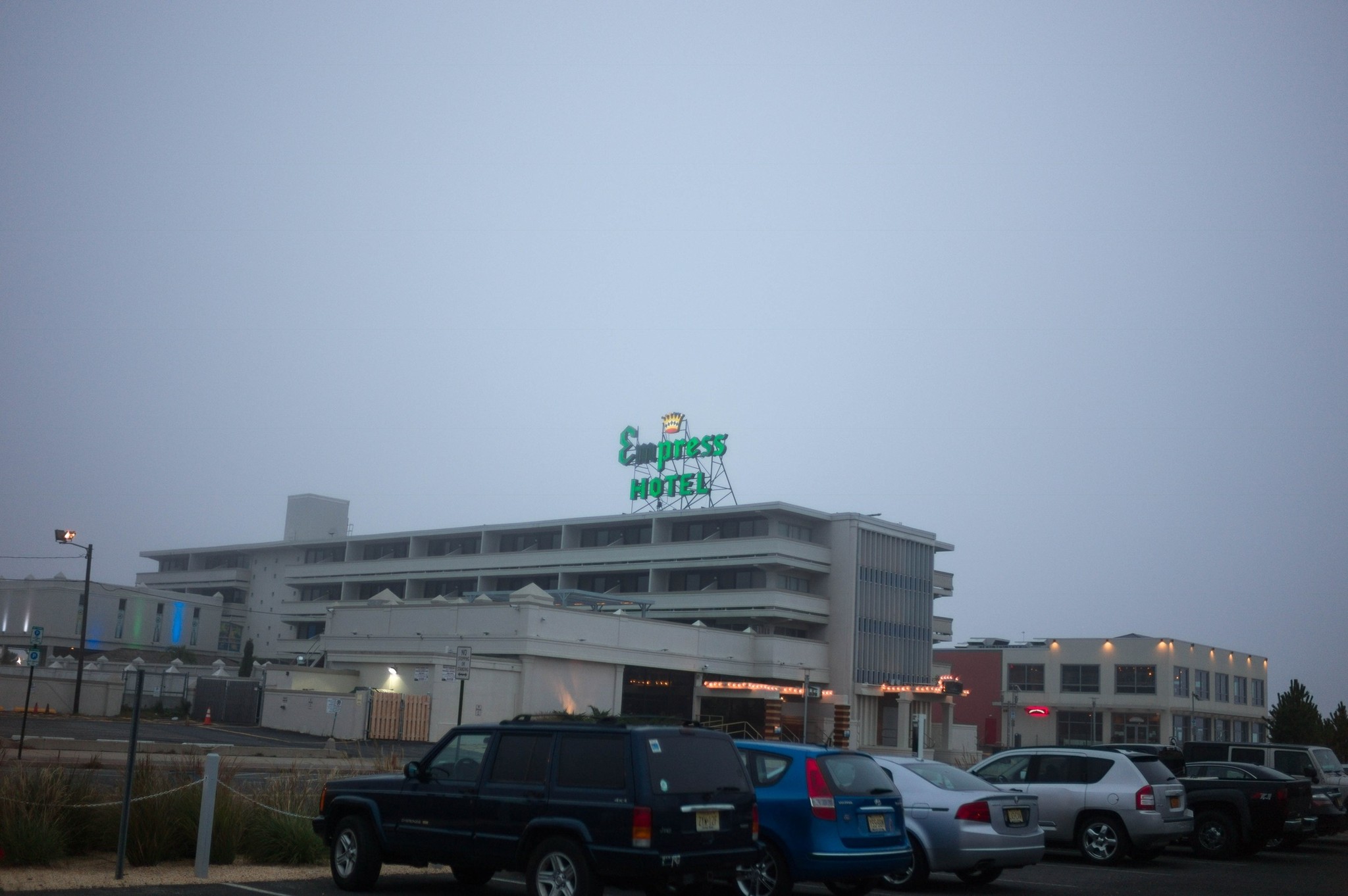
[203,705,214,725]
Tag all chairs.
[1037,762,1058,779]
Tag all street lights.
[54,528,94,715]
[1087,696,1101,745]
[798,667,816,744]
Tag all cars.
[931,746,1196,868]
[733,737,915,896]
[1084,741,1348,861]
[766,755,1046,894]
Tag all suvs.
[312,714,763,896]
